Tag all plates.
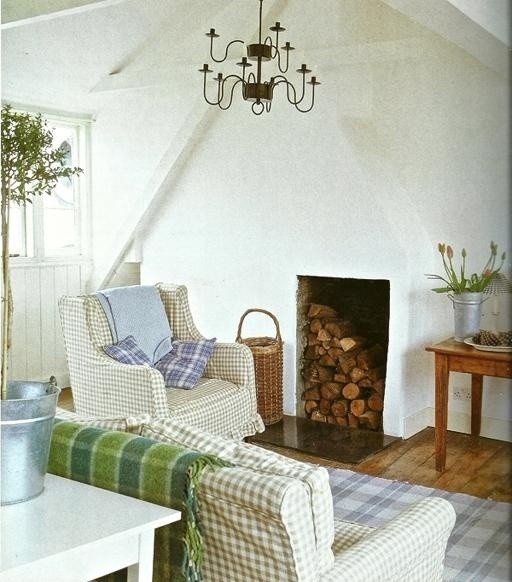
[462,335,512,353]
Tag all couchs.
[50,398,455,582]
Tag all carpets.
[318,465,512,582]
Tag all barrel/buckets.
[448,291,482,342]
[0,375,62,506]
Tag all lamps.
[197,0,322,119]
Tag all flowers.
[418,240,511,297]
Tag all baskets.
[235,309,283,426]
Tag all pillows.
[101,334,217,391]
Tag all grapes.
[472,330,512,347]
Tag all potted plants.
[0,105,84,507]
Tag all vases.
[451,290,483,344]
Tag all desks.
[424,334,512,473]
[0,464,182,582]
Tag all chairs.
[57,283,258,449]
